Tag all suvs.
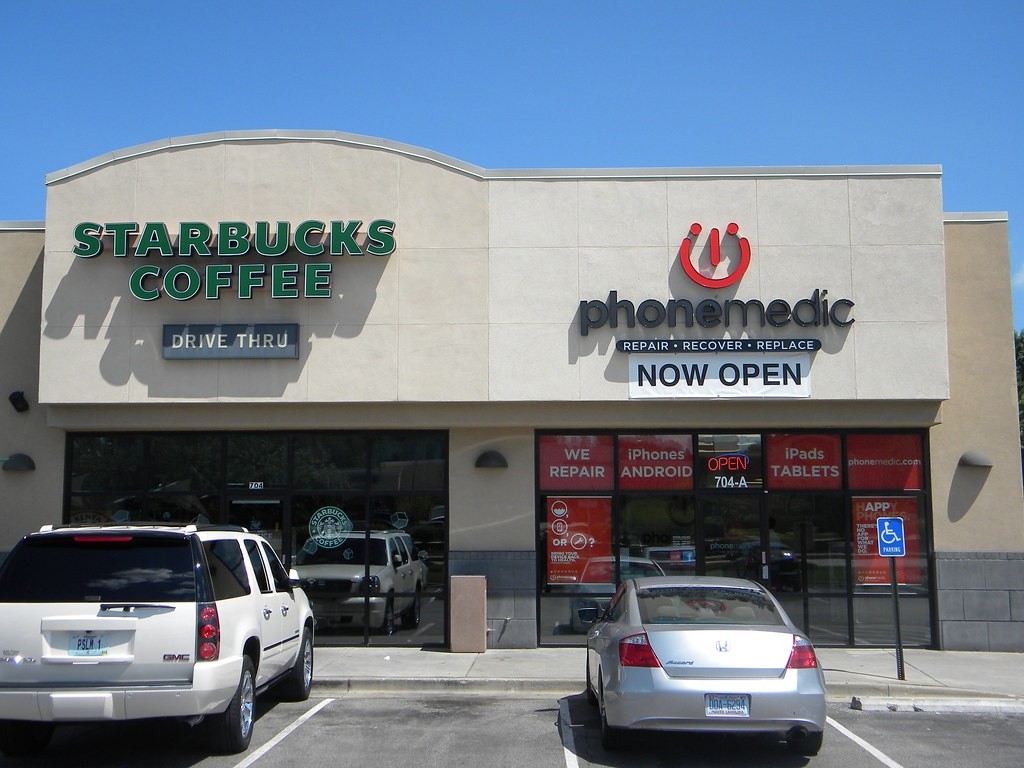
[285,525,431,635]
[0,520,317,754]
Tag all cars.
[577,576,827,756]
[736,541,804,595]
[567,557,668,634]
